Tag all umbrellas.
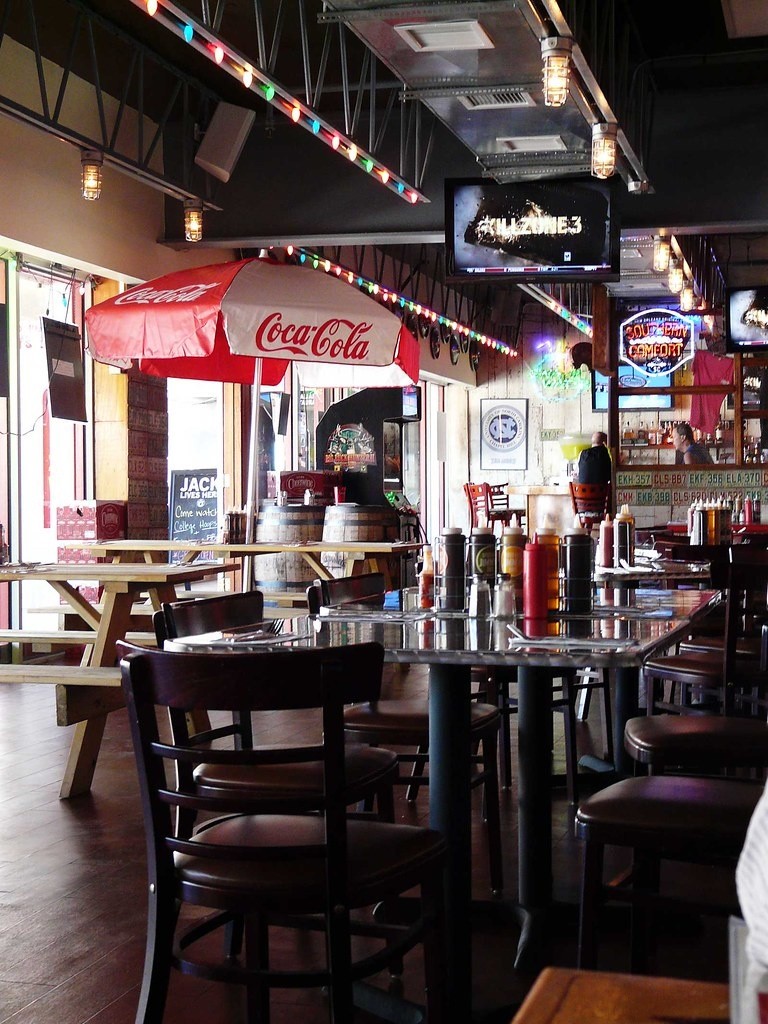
[85,249,420,594]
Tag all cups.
[303,489,314,506]
[334,485,346,503]
[277,490,287,506]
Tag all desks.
[316,589,720,967]
[434,555,711,796]
[163,615,689,1022]
[50,541,425,669]
[1,560,237,799]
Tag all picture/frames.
[479,397,528,472]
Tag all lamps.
[183,198,202,241]
[80,150,103,201]
[591,124,619,179]
[190,102,259,183]
[536,38,576,107]
[667,262,684,293]
[654,236,669,272]
[680,289,693,312]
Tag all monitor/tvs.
[591,363,675,412]
[723,284,768,354]
[445,175,621,285]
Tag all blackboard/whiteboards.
[168,468,220,565]
[729,365,768,407]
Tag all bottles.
[743,376,761,404]
[688,493,761,539]
[223,505,246,544]
[622,421,724,447]
[418,504,635,637]
[744,436,768,464]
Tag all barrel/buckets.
[254,503,326,608]
[322,505,400,605]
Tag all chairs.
[568,482,613,536]
[462,479,525,533]
[116,547,768,1024]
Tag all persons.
[574,432,607,529]
[673,423,714,464]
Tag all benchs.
[0,630,171,688]
[27,587,329,620]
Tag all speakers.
[194,101,257,182]
